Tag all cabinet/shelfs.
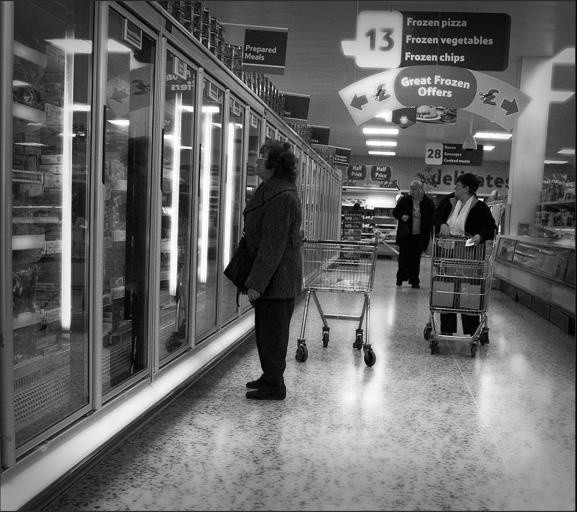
[339,203,399,260]
[486,195,576,336]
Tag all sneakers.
[396,278,420,289]
[245,371,286,401]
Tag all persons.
[436,173,498,336]
[223,140,303,401]
[392,180,436,289]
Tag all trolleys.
[295,229,379,367]
[423,225,490,357]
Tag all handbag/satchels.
[222,236,259,295]
